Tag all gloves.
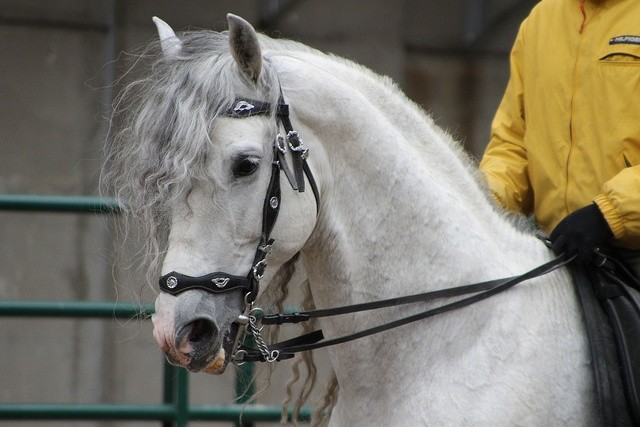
[549,203,613,256]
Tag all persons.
[475,1,639,277]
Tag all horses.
[96,15,604,426]
